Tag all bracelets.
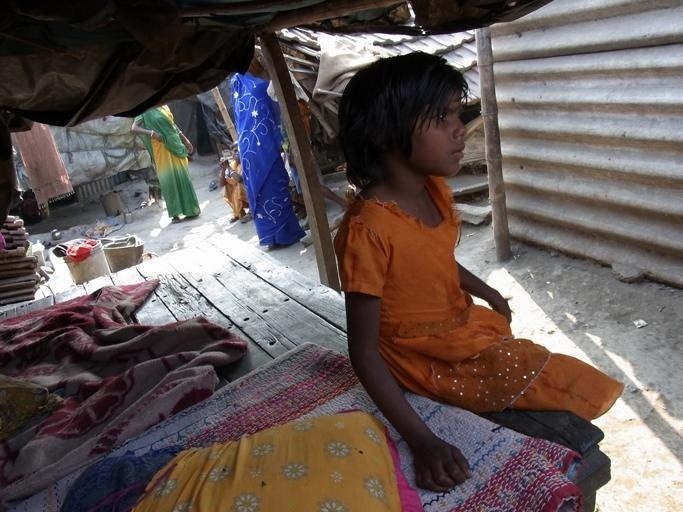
[179,131,182,134]
[230,170,236,176]
[151,130,153,136]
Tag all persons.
[230,72,307,246]
[332,51,624,493]
[219,141,252,224]
[131,104,201,222]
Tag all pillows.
[59,407,426,511]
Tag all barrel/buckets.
[62,240,111,287]
[102,238,145,275]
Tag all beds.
[1,233,611,512]
